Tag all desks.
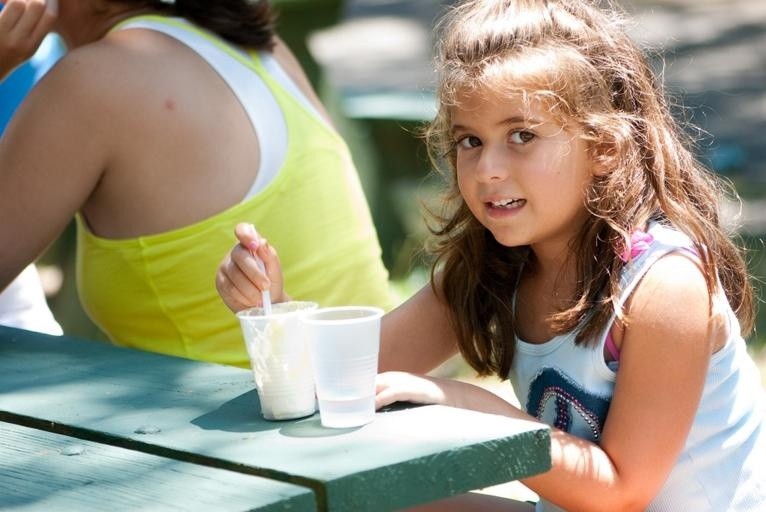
[0,325,551,512]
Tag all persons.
[213,0,763,508]
[0,1,394,380]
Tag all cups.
[234,301,384,429]
